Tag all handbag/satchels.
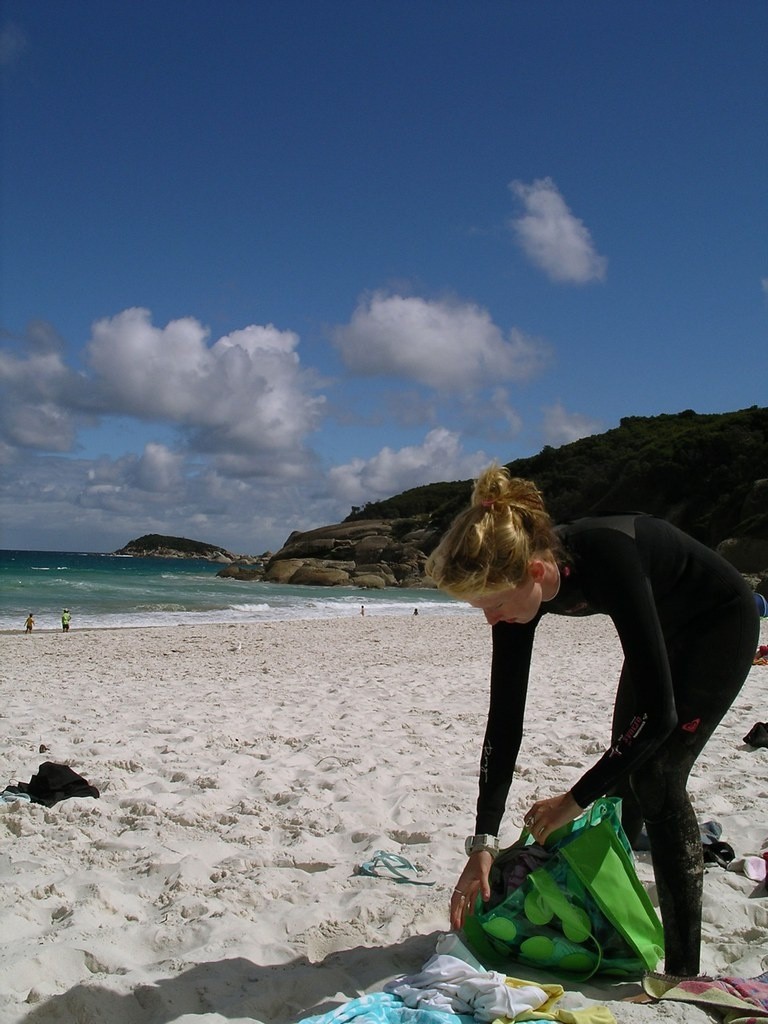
[462,797,666,984]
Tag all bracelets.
[465,834,500,859]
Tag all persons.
[62,608,72,632]
[24,613,34,634]
[361,605,365,617]
[423,464,760,1003]
[414,608,419,615]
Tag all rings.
[454,889,462,893]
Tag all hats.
[63,608,69,612]
